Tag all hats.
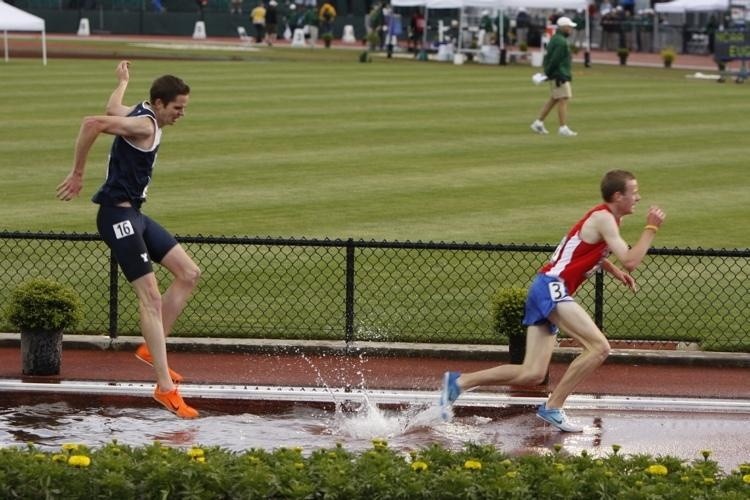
[532,72,548,85]
[557,16,577,27]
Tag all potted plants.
[366,31,379,50]
[616,46,629,65]
[1,277,79,379]
[516,40,528,59]
[321,32,333,50]
[658,46,675,68]
[493,282,549,385]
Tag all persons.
[528,16,582,138]
[440,169,669,435]
[53,58,205,420]
[155,1,534,59]
[601,6,668,54]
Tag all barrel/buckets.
[530,52,544,67]
[452,53,464,65]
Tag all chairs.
[236,26,254,47]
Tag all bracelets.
[644,224,658,233]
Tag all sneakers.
[557,125,578,137]
[530,118,550,135]
[536,403,582,434]
[134,344,184,384]
[153,384,198,420]
[440,372,461,423]
